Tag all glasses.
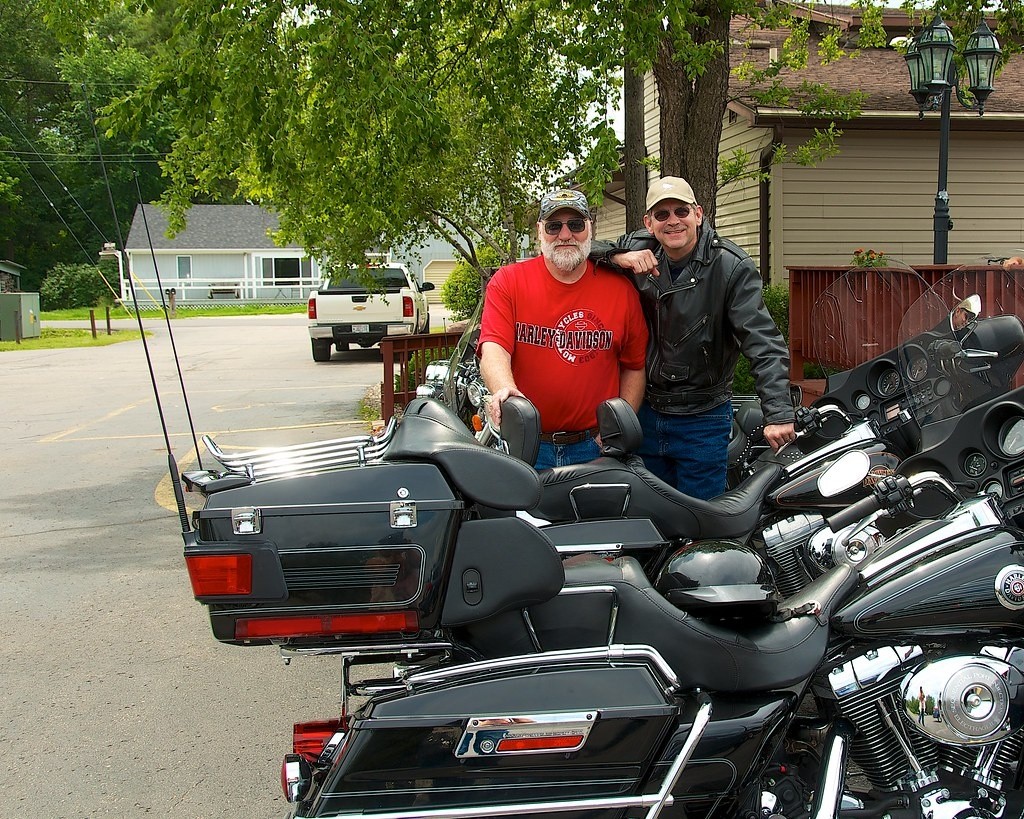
[542,218,585,235]
[654,207,693,221]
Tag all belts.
[540,427,600,446]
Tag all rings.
[785,438,791,442]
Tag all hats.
[646,176,697,213]
[539,189,592,221]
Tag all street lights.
[905,9,1002,264]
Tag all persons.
[587,176,796,499]
[475,189,645,470]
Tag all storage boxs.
[292,667,684,819]
[542,517,673,582]
[184,459,467,646]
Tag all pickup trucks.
[306,253,434,363]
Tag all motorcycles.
[176,312,1024,819]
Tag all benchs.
[207,282,240,299]
